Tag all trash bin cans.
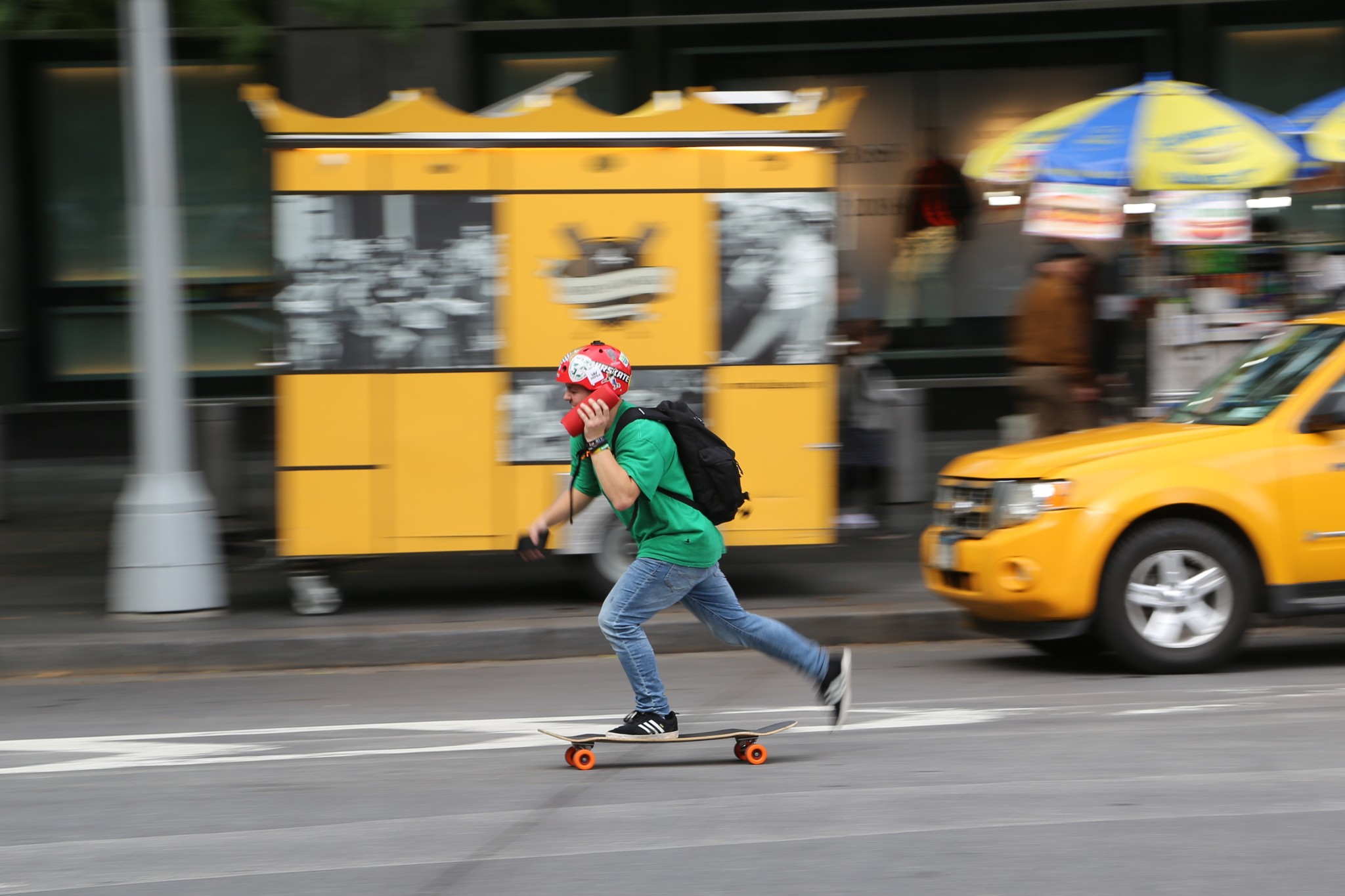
[851,384,935,507]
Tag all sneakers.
[606,709,678,741]
[819,647,851,726]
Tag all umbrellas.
[956,76,1334,297]
[1284,83,1345,165]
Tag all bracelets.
[581,436,611,459]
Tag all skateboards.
[537,719,799,771]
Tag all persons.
[1016,242,1119,373]
[518,339,852,741]
[895,126,979,242]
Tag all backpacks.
[581,401,750,526]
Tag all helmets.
[556,340,632,395]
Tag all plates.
[1286,252,1345,307]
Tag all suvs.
[919,310,1345,676]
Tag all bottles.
[561,383,620,438]
